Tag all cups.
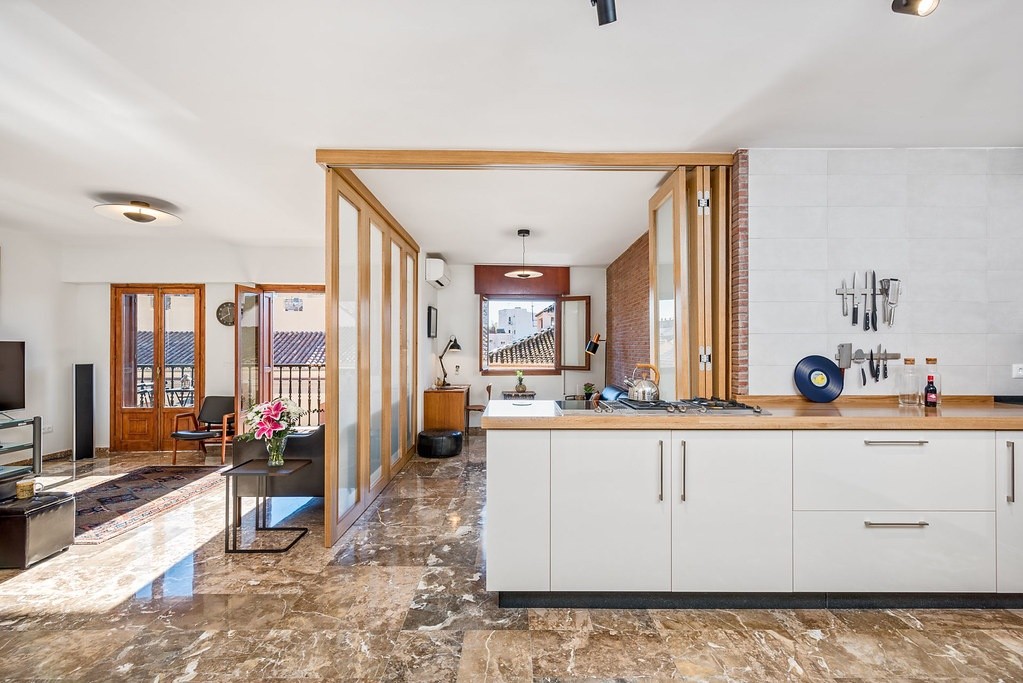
[15,480,45,499]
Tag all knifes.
[852,270,860,326]
[874,344,882,381]
[872,270,878,331]
[865,272,871,330]
[869,349,875,376]
[843,280,848,316]
[883,349,887,378]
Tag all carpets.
[71,465,233,546]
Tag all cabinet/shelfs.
[996,431,1023,594]
[0,416,42,486]
[792,431,995,594]
[551,428,794,592]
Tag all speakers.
[72,363,95,461]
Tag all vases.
[264,434,287,466]
[516,381,526,393]
[584,390,592,401]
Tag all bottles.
[924,375,937,408]
[919,357,942,404]
[898,358,919,405]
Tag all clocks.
[216,302,238,326]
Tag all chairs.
[466,383,492,437]
[170,396,237,464]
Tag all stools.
[418,429,463,458]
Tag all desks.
[0,491,77,569]
[424,383,471,437]
[502,391,536,401]
[163,389,195,407]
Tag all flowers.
[514,371,529,383]
[584,384,594,393]
[245,396,308,441]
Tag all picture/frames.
[427,306,437,338]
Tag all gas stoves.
[598,395,754,415]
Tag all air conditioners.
[425,257,451,289]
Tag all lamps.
[505,230,544,279]
[94,200,183,229]
[437,335,461,386]
[586,332,607,355]
[892,0,940,17]
[587,0,617,25]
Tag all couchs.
[584,385,630,401]
[227,423,324,528]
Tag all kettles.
[623,363,661,401]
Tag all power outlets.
[1013,364,1023,379]
[42,425,54,434]
[454,365,460,375]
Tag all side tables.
[222,459,313,553]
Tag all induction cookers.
[555,400,628,410]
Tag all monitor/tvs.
[0,341,25,411]
